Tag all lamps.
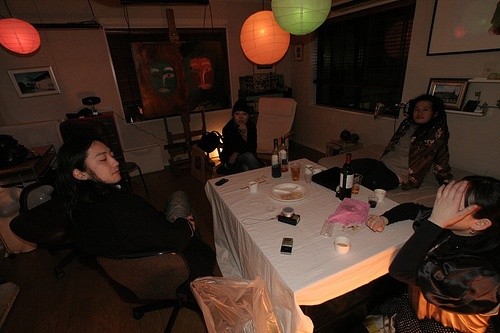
[240,0,292,66]
[0,1,41,54]
[274,0,333,36]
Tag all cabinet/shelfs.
[445,79,500,117]
[66,112,123,164]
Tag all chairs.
[255,99,301,164]
[96,250,206,333]
[10,182,78,279]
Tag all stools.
[120,162,147,193]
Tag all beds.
[320,150,477,216]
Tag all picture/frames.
[295,43,304,61]
[8,66,62,98]
[105,26,234,124]
[426,76,470,108]
[253,63,275,75]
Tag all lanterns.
[270,0,332,35]
[239,10,290,66]
[0,18,41,55]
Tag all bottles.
[270,137,282,178]
[280,134,288,172]
[340,153,355,201]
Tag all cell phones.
[281,237,294,255]
[215,179,228,186]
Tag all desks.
[0,144,58,188]
[204,158,418,332]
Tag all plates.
[271,182,309,203]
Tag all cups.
[290,162,301,181]
[350,172,363,194]
[334,237,350,254]
[367,195,378,208]
[374,189,387,203]
[249,181,259,193]
[304,164,313,184]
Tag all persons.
[218,97,262,172]
[364,175,500,333]
[8,134,216,302]
[343,94,453,194]
